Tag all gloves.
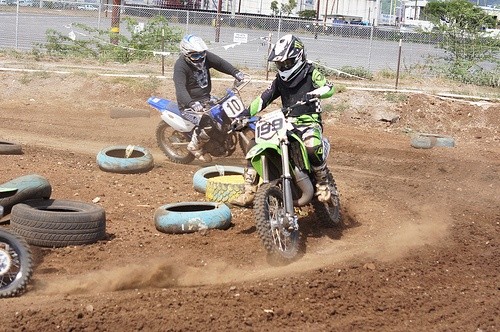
[231,110,251,132]
[297,92,320,105]
[190,101,203,112]
[235,71,244,82]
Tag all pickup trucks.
[78,4,98,11]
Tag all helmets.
[268,34,307,82]
[179,34,208,71]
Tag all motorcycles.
[148,73,263,163]
[226,95,344,265]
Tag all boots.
[187,132,212,164]
[312,165,331,202]
[242,183,258,205]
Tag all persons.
[173,34,244,163]
[228,34,334,202]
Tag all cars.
[1,0,80,11]
